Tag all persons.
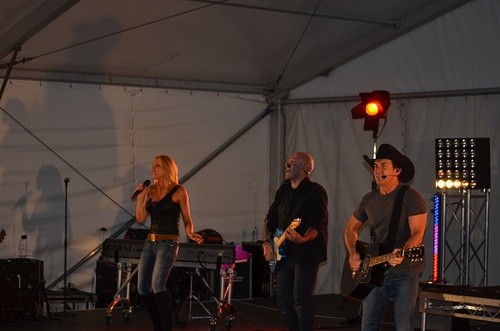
[136,155,204,331]
[344,143,427,331]
[262,151,328,331]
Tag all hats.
[363,144,415,184]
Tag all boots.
[155,290,176,331]
[139,294,163,331]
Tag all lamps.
[434,136,492,286]
[352,90,392,189]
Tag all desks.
[419,284,500,331]
[240,240,270,292]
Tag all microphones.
[382,175,396,179]
[131,180,150,199]
[287,164,307,174]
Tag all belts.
[147,233,179,242]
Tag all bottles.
[253,226,258,243]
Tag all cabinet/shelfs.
[0,258,46,319]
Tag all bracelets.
[263,240,271,246]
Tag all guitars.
[266,216,301,273]
[339,237,425,302]
